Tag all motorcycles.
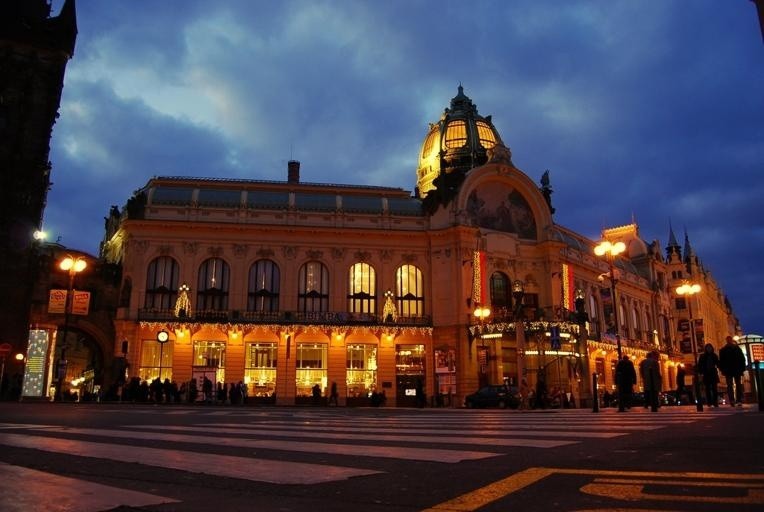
[603,390,617,407]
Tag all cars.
[462,383,521,408]
[616,385,729,406]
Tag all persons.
[638,353,660,412]
[615,355,636,412]
[699,343,719,408]
[328,380,338,406]
[676,364,685,394]
[532,375,544,409]
[719,336,746,407]
[520,379,529,408]
[312,384,320,402]
[123,376,243,404]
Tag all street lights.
[54,250,88,401]
[593,237,628,361]
[674,278,704,368]
